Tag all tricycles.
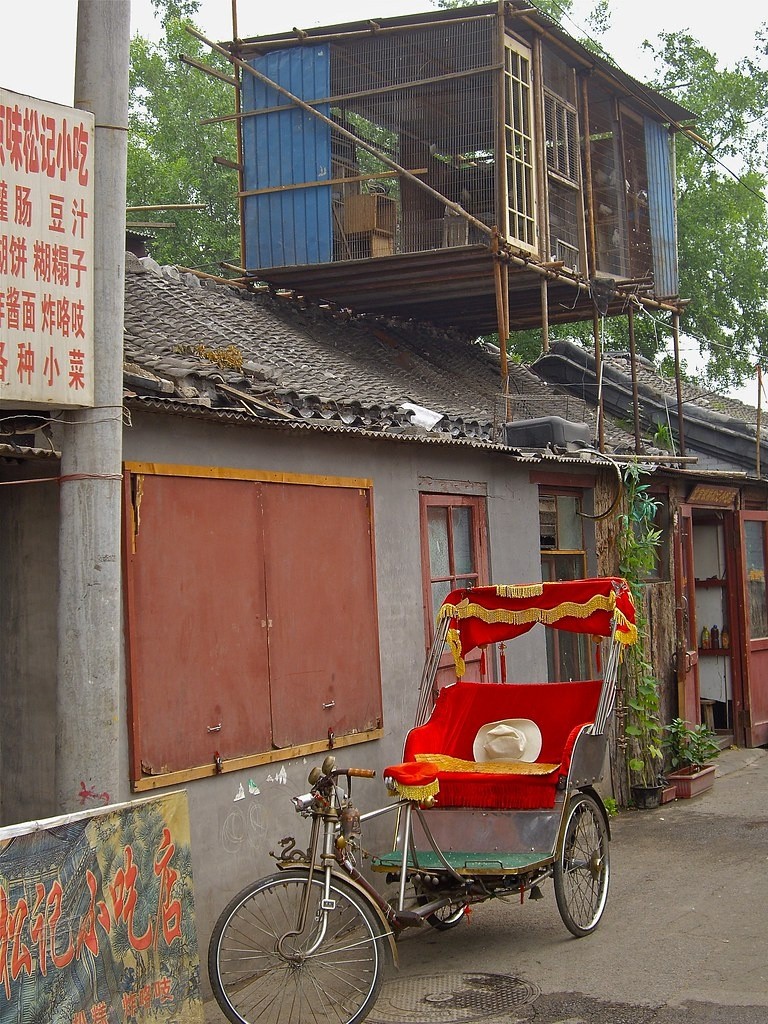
[208,577,657,1024]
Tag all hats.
[472,718,542,763]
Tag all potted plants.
[622,455,721,807]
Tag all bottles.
[701,625,711,650]
[721,624,729,650]
[710,624,719,649]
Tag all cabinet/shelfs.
[329,113,397,260]
[551,186,647,275]
[695,578,728,655]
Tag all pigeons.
[425,141,648,247]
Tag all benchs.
[400,679,603,808]
[701,699,716,734]
